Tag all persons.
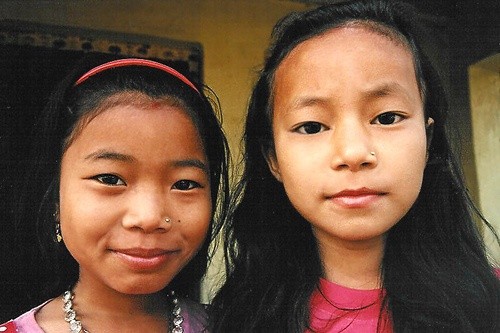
[2,50,236,333]
[201,0,500,333]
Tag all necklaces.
[61,284,186,333]
